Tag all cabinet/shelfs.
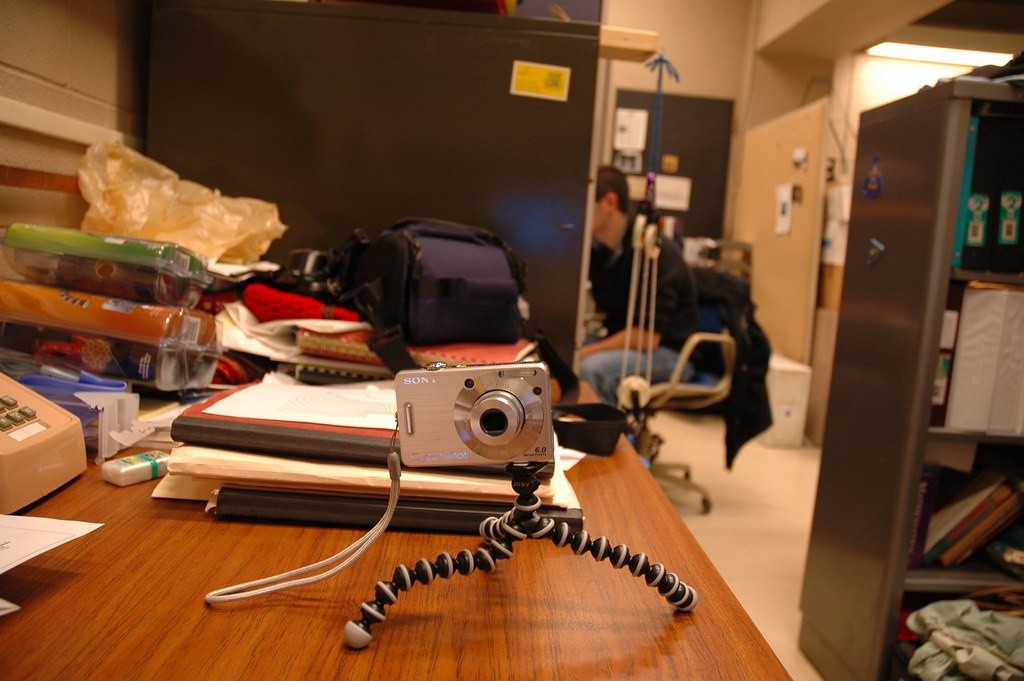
[797,81,1023,681]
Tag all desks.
[0,381,795,681]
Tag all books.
[167,380,586,537]
[906,470,1021,569]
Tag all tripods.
[341,463,699,650]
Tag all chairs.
[636,241,774,514]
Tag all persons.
[578,165,698,406]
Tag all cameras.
[394,360,555,467]
